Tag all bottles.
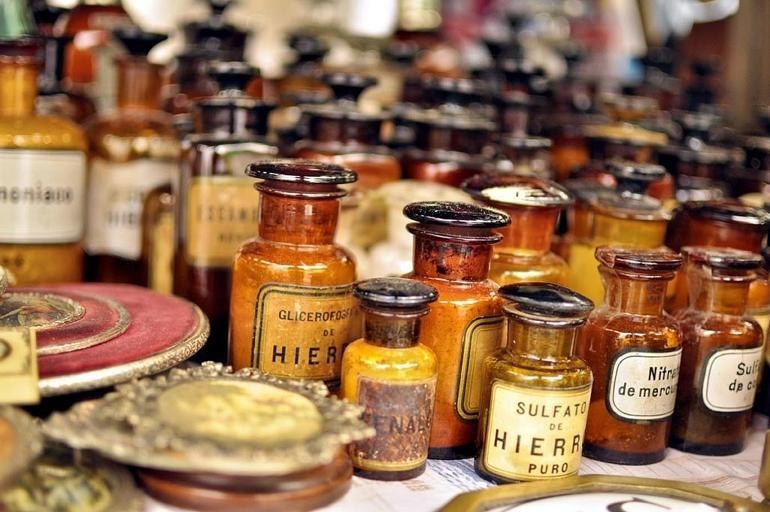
[393,104,494,185]
[185,5,715,109]
[336,277,440,481]
[580,244,686,464]
[677,193,770,337]
[0,35,89,281]
[668,244,762,456]
[227,157,354,393]
[567,165,672,302]
[477,280,596,483]
[82,29,181,282]
[404,197,510,461]
[187,61,279,314]
[287,76,401,198]
[658,115,736,189]
[476,137,575,286]
[576,86,672,200]
